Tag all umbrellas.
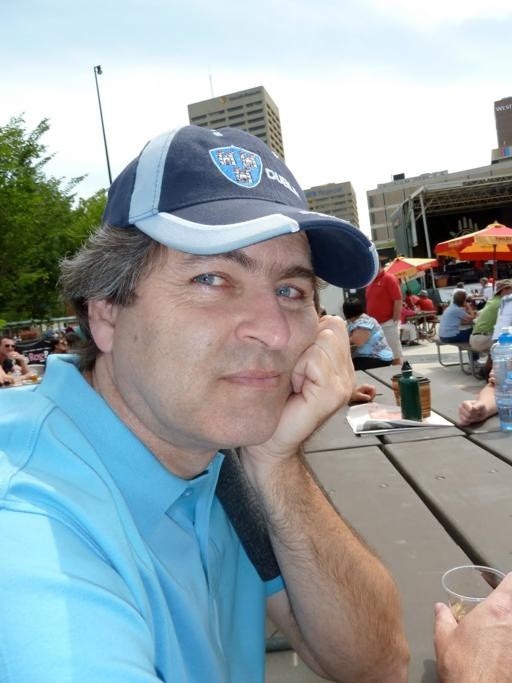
[434,220,512,291]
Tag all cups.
[392,373,401,406]
[441,565,506,623]
[415,376,431,418]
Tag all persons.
[0,124,512,683]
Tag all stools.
[396,300,512,377]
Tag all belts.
[473,332,486,334]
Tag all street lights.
[92,65,114,187]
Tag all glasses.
[5,344,15,347]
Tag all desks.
[237,366,512,679]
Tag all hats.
[101,124,380,288]
[417,289,430,296]
[494,278,512,294]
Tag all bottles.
[6,370,40,388]
[398,361,423,423]
[490,332,512,432]
